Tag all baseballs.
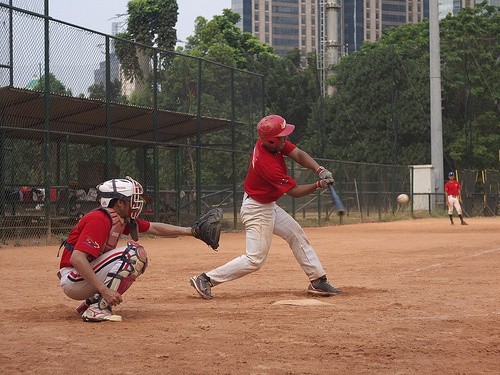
[397,194,409,206]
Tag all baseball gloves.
[191,207,220,251]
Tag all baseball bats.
[327,180,347,215]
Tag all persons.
[190,115,344,300]
[57,176,222,322]
[445,172,469,225]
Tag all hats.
[257,115,295,150]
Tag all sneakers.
[75,302,88,314]
[189,272,215,300]
[82,303,122,322]
[307,275,344,296]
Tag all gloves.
[316,164,334,180]
[317,178,335,189]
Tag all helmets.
[96,175,145,227]
